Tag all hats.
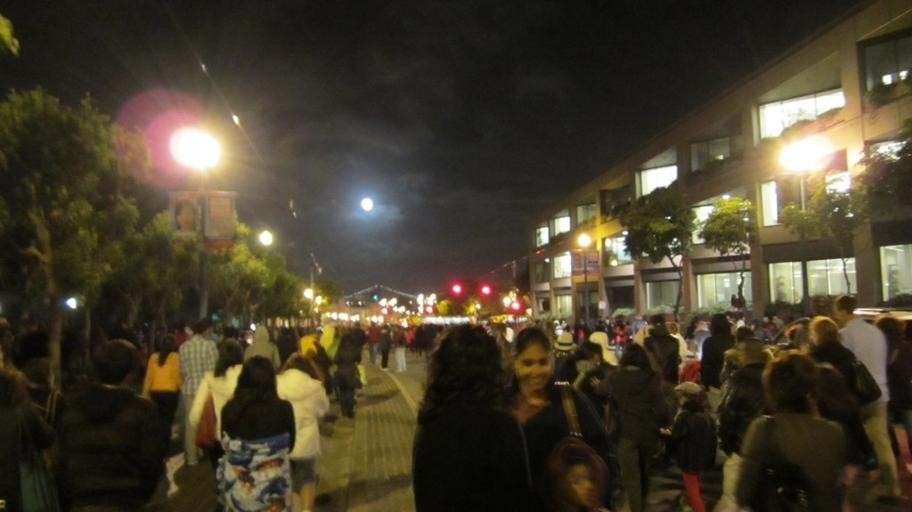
[554,333,577,351]
[674,382,702,395]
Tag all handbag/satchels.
[194,396,218,452]
[750,464,815,511]
[651,429,677,469]
[547,435,610,510]
[17,447,60,511]
[843,362,880,407]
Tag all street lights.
[577,234,599,339]
[776,144,821,318]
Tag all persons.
[1,296,912,511]
